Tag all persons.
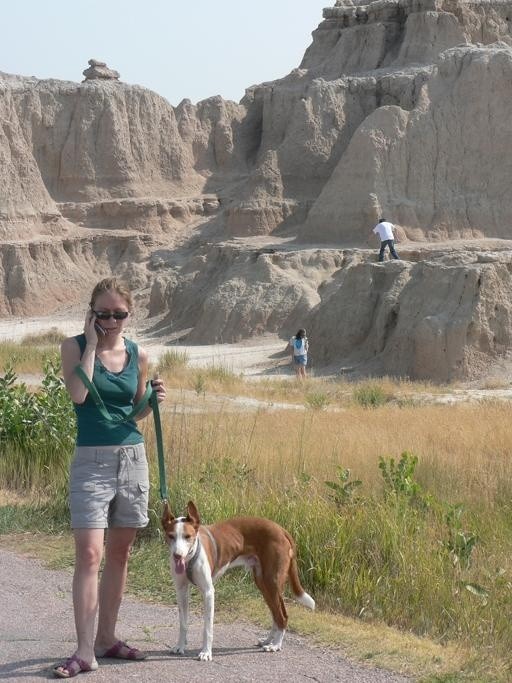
[292,328,309,379]
[52,276,165,677]
[371,218,400,261]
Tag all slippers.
[53,655,98,678]
[95,641,148,660]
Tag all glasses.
[92,311,128,320]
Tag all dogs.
[160,499,316,662]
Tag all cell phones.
[89,317,108,336]
